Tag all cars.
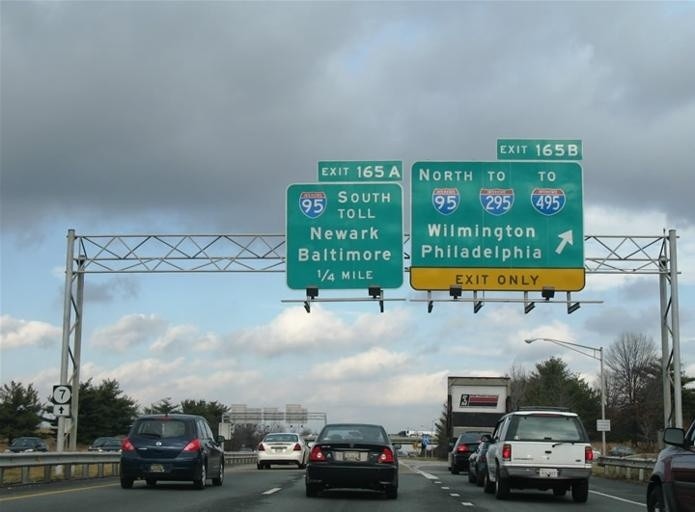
[88,413,226,490]
[4,436,49,453]
[646,416,695,512]
[256,423,401,500]
[447,407,593,502]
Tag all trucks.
[447,376,511,440]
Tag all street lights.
[525,336,606,457]
